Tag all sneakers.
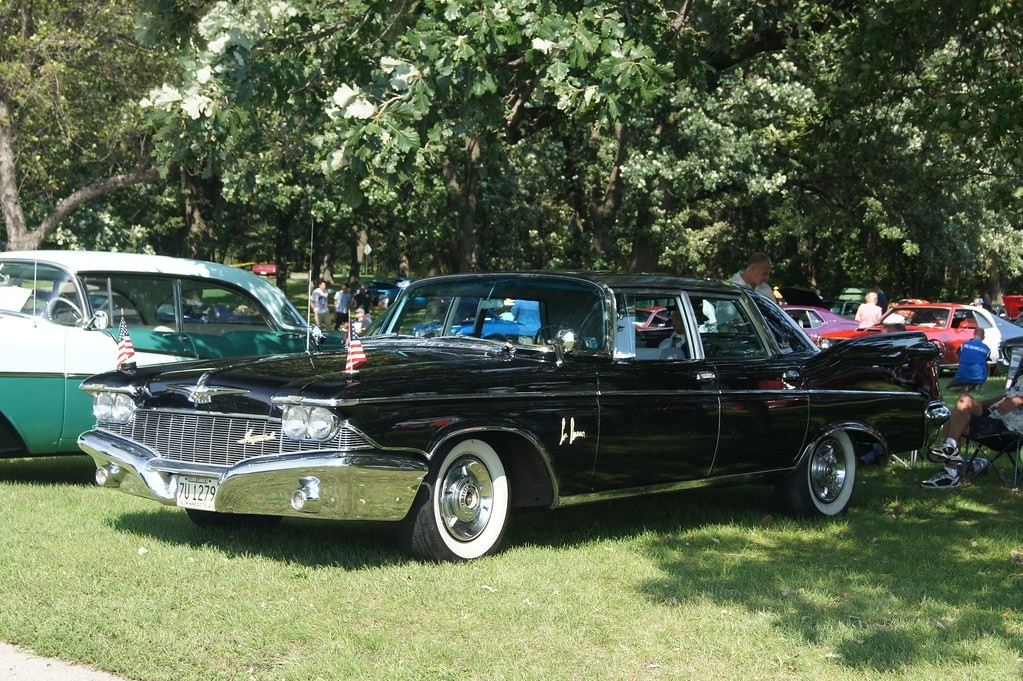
[927,444,965,467]
[921,469,962,490]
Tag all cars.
[764,306,875,343]
[0,248,356,460]
[359,273,436,311]
[820,302,1023,374]
[411,297,540,346]
[252,259,280,277]
[47,291,264,330]
[614,304,681,332]
[75,270,950,566]
[776,282,1023,328]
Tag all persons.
[343,308,372,341]
[352,283,375,315]
[852,290,883,331]
[918,371,1023,490]
[872,285,891,313]
[970,297,983,309]
[881,301,907,324]
[943,327,992,392]
[332,283,351,330]
[644,294,718,359]
[310,282,333,329]
[713,251,777,334]
[910,309,937,326]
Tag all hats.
[668,296,710,325]
[354,307,365,314]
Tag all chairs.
[553,306,753,359]
[957,347,1023,487]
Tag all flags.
[115,315,136,368]
[340,314,367,375]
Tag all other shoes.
[964,384,986,394]
